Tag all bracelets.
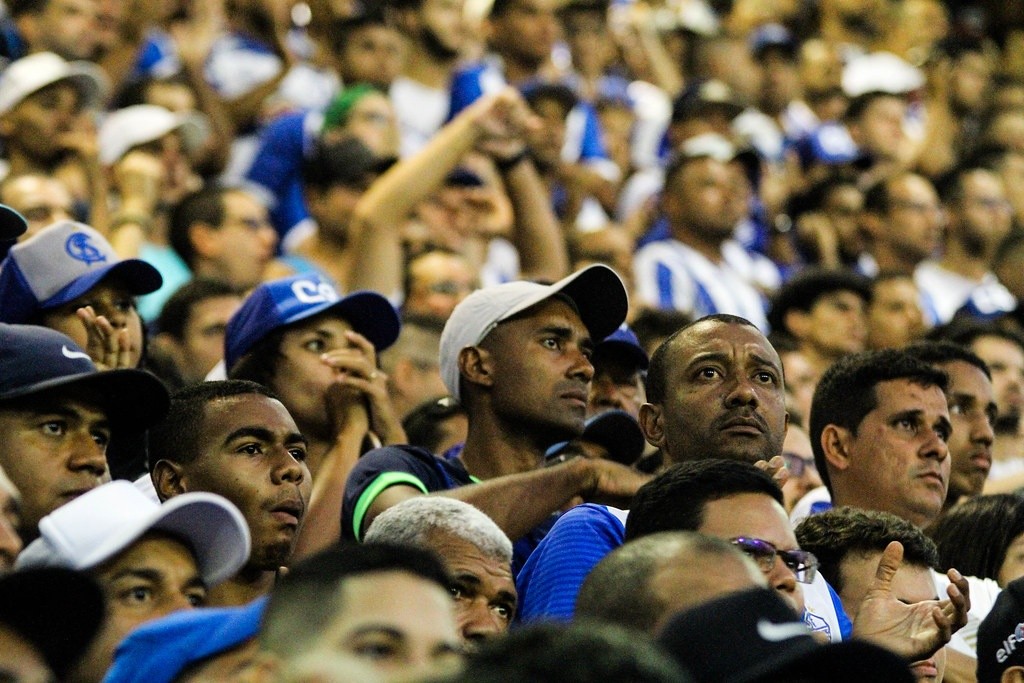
[494,146,531,173]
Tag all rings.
[367,373,377,381]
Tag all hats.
[13,479,252,588]
[438,264,628,403]
[97,104,213,168]
[0,220,162,323]
[593,319,650,370]
[223,275,400,375]
[0,323,170,430]
[977,576,1024,679]
[0,204,27,257]
[544,409,645,466]
[659,586,916,683]
[0,51,114,123]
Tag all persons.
[0,205,1024,683]
[0,0,1024,518]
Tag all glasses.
[728,535,821,586]
[779,452,816,475]
